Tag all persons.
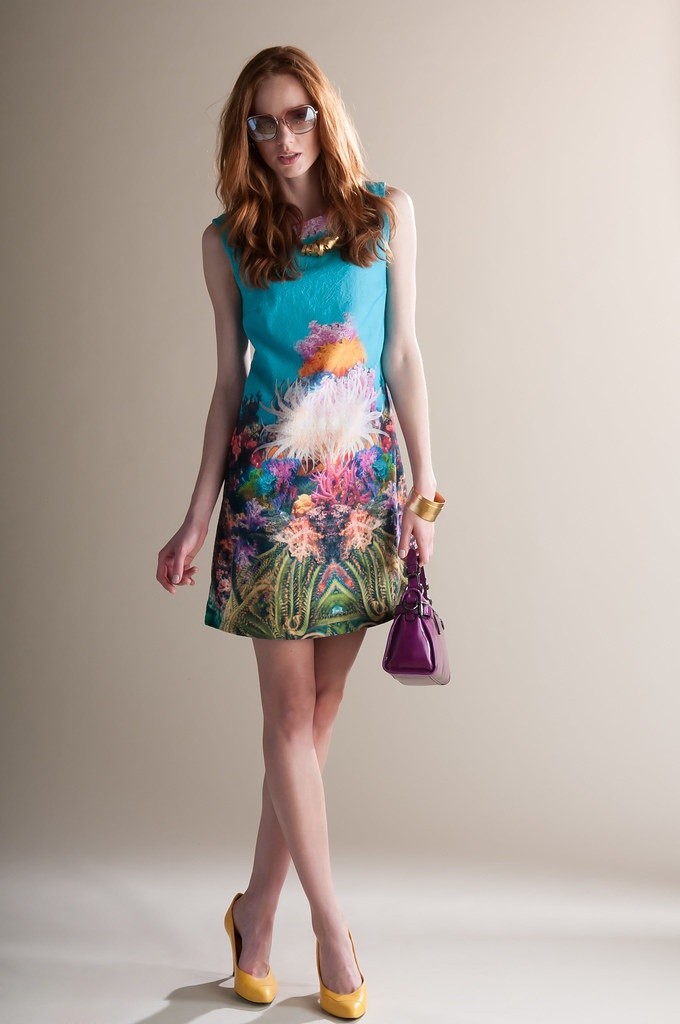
[154,46,445,1020]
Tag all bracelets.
[407,487,444,522]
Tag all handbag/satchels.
[381,548,451,685]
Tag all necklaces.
[299,234,340,258]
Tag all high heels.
[224,892,277,1003]
[315,927,367,1019]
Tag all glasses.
[247,104,319,143]
